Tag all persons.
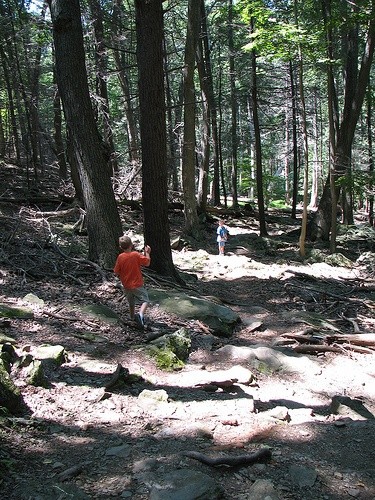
[113,236,151,330]
[216,220,228,256]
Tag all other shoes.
[137,313,144,327]
[131,314,136,321]
